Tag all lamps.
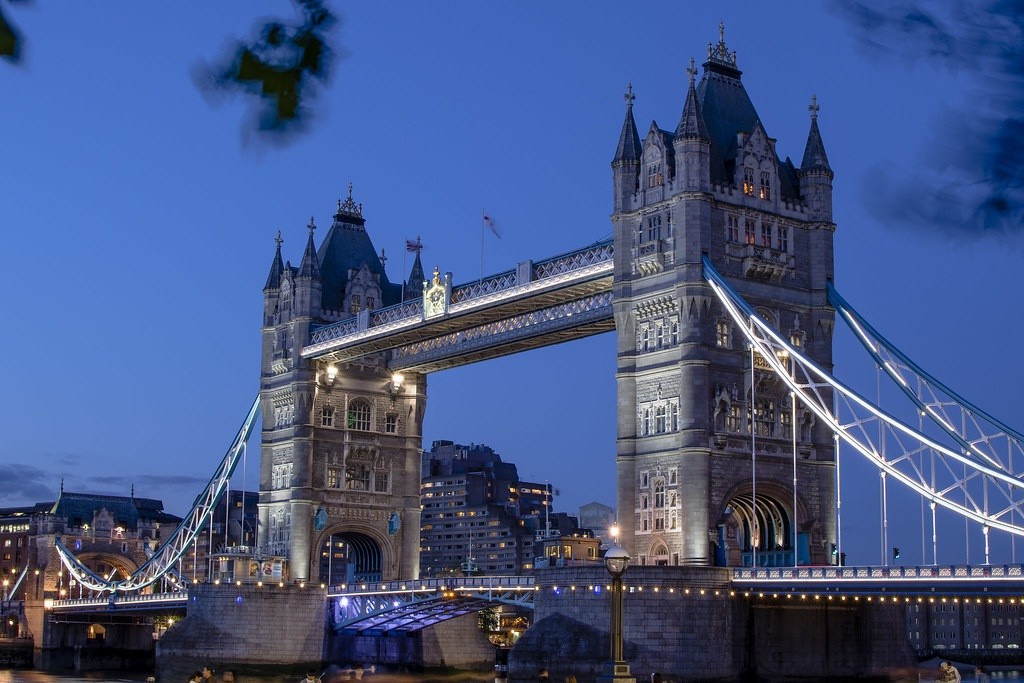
[389,372,405,395]
[324,363,339,385]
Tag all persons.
[975,666,991,683]
[190,664,662,683]
[936,662,961,683]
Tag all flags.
[482,213,503,238]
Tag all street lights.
[604,544,632,683]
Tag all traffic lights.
[830,542,837,555]
[893,547,900,559]
